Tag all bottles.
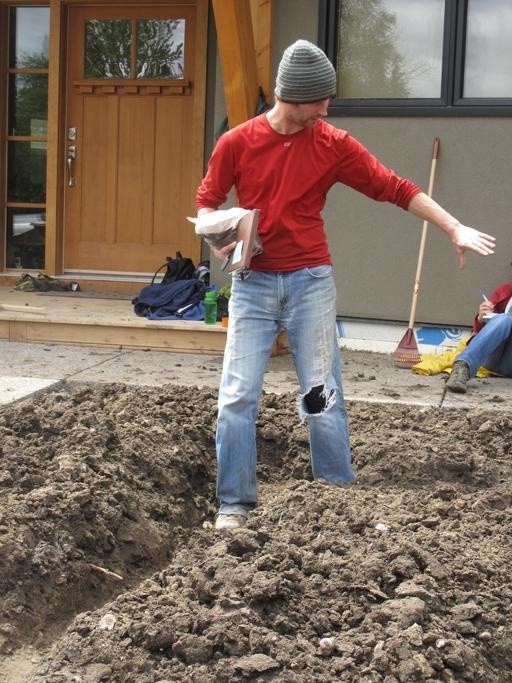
[204,292,217,324]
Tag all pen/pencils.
[480,288,488,301]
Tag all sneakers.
[446,360,470,393]
[216,514,247,529]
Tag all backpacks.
[151,251,195,285]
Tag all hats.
[275,39,337,103]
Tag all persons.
[195,38,497,533]
[445,283,512,394]
